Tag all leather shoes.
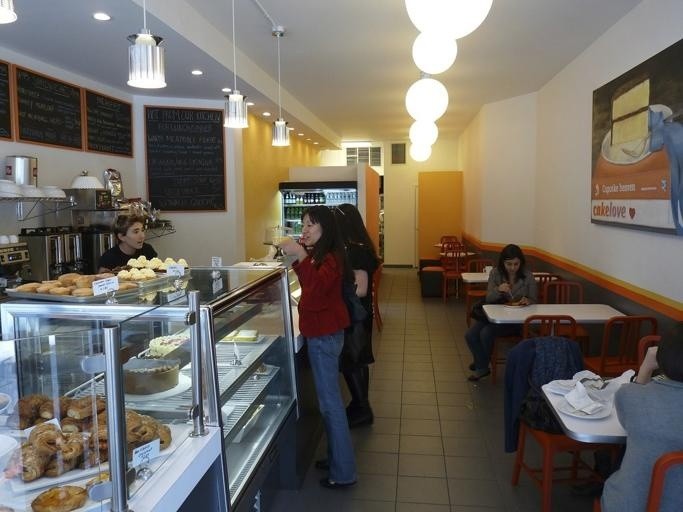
[315,459,331,470]
[468,368,490,381]
[320,475,357,488]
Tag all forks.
[621,108,683,158]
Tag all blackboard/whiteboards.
[0,60,14,141]
[11,62,83,152]
[144,103,227,212]
[83,87,134,159]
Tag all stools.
[419,254,445,298]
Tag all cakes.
[123,358,181,395]
[146,333,190,359]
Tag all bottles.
[284,192,357,235]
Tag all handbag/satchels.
[471,300,486,321]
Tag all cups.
[0,235,9,244]
[483,265,493,275]
[9,235,19,243]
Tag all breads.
[3,393,172,482]
[222,329,259,342]
[17,254,189,298]
[31,483,89,512]
[611,73,650,147]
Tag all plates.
[7,428,92,439]
[556,393,613,419]
[252,364,275,376]
[219,335,267,345]
[548,379,577,396]
[503,305,526,308]
[601,104,673,166]
[0,392,12,415]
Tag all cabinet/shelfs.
[280,180,359,238]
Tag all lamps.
[126,0,167,90]
[405,0,494,164]
[222,0,250,129]
[271,26,290,148]
[1,1,17,25]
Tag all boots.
[342,365,373,428]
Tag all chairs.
[372,263,384,332]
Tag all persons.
[337,204,379,427]
[600,320,682,512]
[98,216,157,350]
[276,206,358,492]
[465,245,539,383]
